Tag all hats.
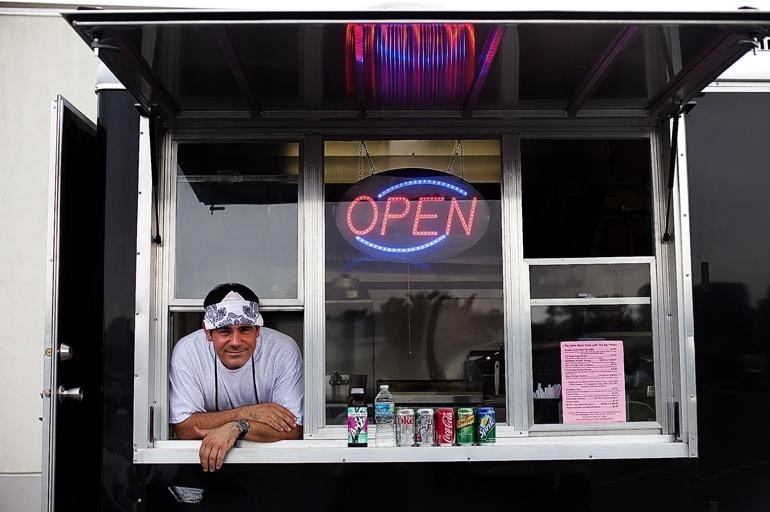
[197,290,271,332]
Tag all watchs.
[236,419,249,441]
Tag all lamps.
[326,271,372,305]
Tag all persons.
[168,282,304,473]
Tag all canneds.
[395,406,496,448]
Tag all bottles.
[374,384,396,446]
[346,385,369,446]
